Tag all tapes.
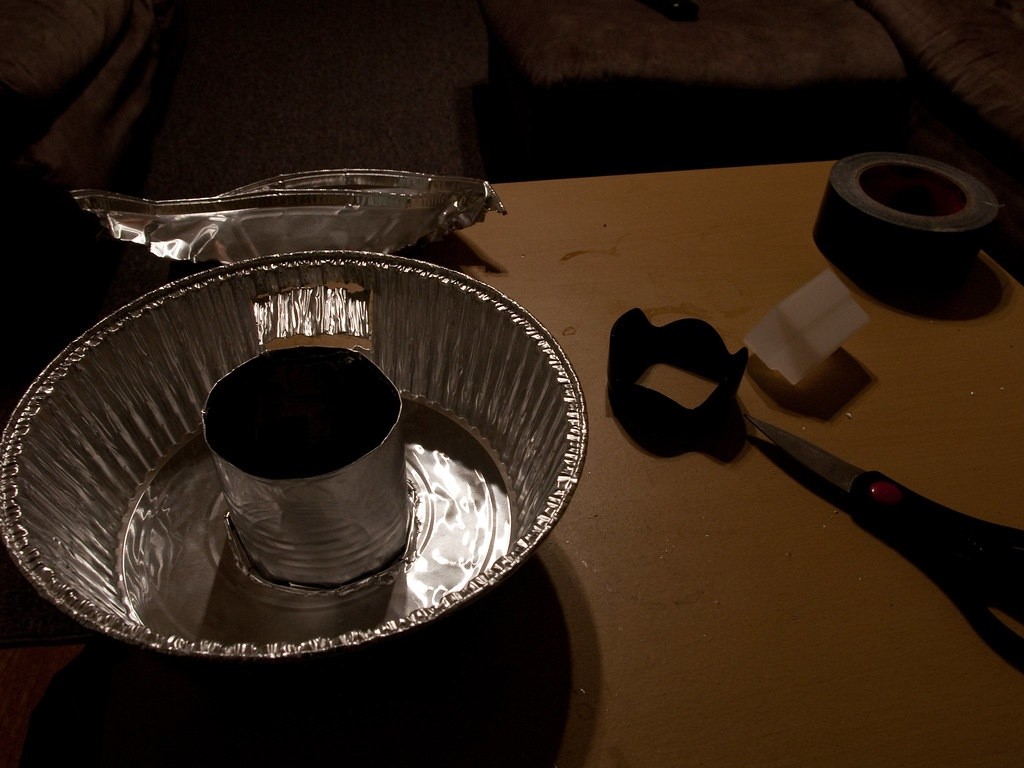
[811,151,1000,290]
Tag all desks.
[1,160,1024,768]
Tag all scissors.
[742,412,1024,675]
